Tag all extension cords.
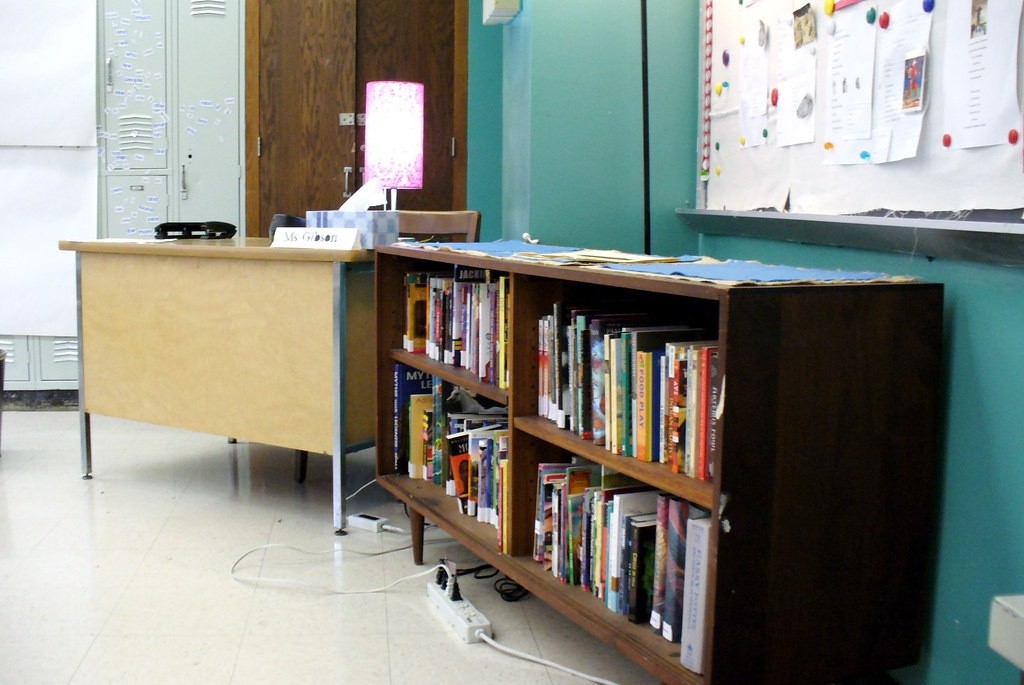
[427,581,493,644]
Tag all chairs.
[395,209,481,242]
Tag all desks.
[59,237,375,536]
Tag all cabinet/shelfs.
[0,0,245,391]
[373,245,946,685]
[245,0,468,243]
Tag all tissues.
[306,176,398,249]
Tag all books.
[532,301,718,675]
[393,270,510,555]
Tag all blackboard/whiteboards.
[676,0,1024,267]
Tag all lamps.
[363,80,425,209]
